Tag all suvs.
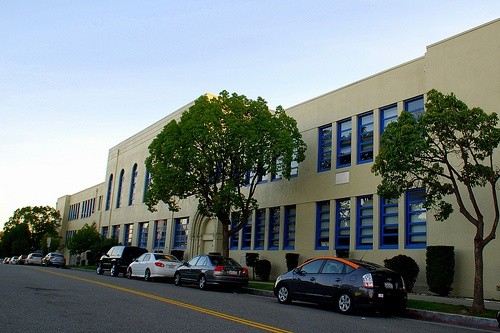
[95,245,150,277]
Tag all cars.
[24,252,44,266]
[0,254,27,265]
[272,256,408,317]
[41,252,66,269]
[173,253,250,292]
[126,252,184,281]
